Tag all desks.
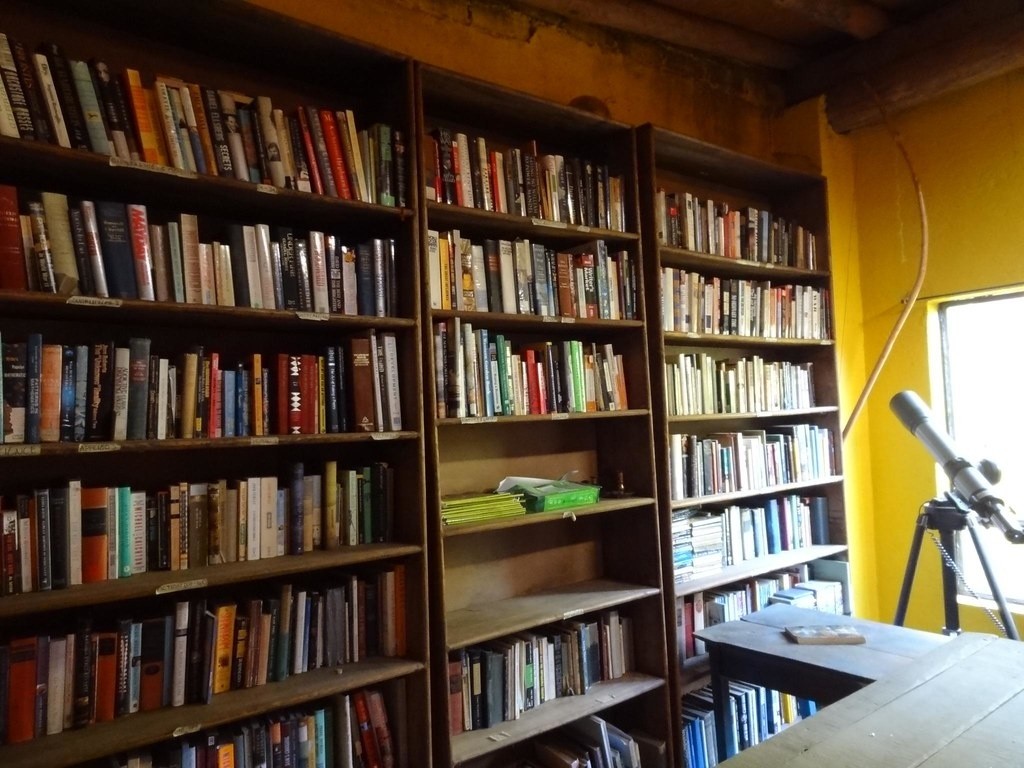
[708,630,1024,768]
[692,603,955,763]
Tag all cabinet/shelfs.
[0,0,435,768]
[637,120,860,768]
[413,56,682,768]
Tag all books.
[0,28,410,768]
[418,125,865,768]
